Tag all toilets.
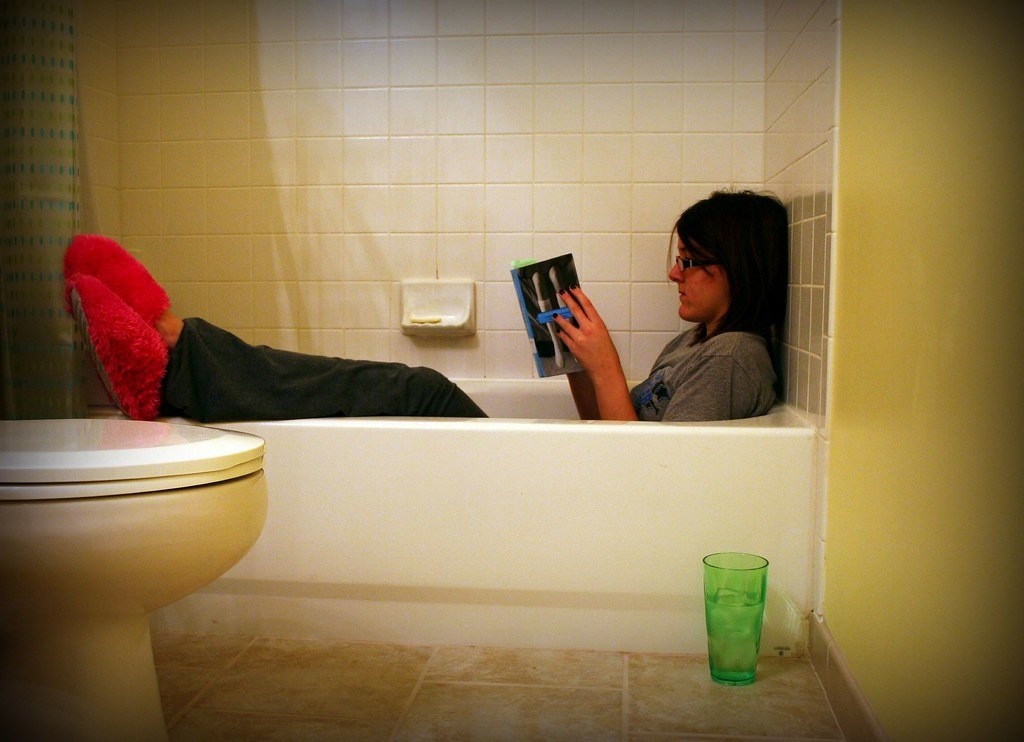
[2,410,274,741]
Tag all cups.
[703,552,769,686]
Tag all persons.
[61,188,791,423]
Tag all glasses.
[676,256,723,272]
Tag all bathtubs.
[102,369,822,656]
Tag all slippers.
[63,235,172,327]
[65,272,169,421]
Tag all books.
[511,254,589,378]
